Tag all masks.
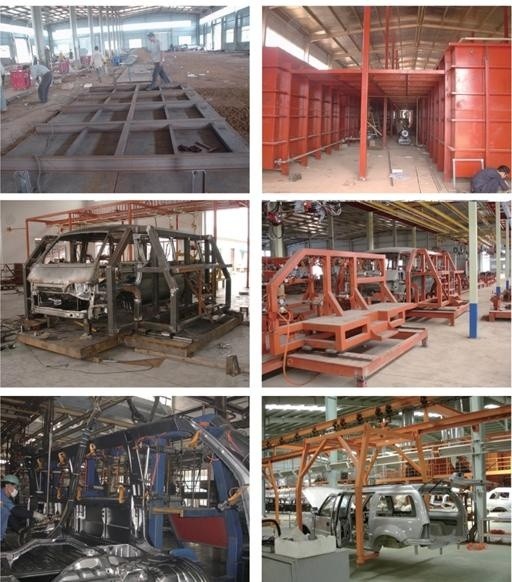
[8,485,19,498]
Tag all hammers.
[196,141,218,152]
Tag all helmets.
[1,474,19,485]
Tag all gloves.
[32,511,48,521]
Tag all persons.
[20,64,52,102]
[0,63,9,113]
[471,165,511,194]
[89,45,107,83]
[30,54,38,66]
[0,474,48,543]
[59,48,74,62]
[144,32,171,91]
